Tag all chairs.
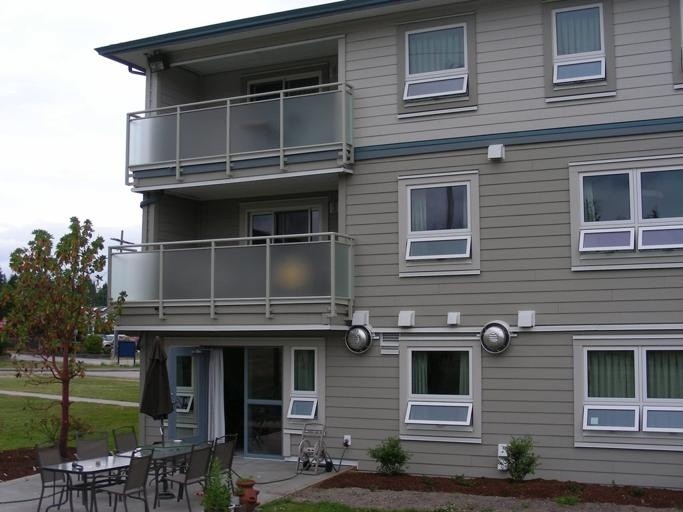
[37,425,239,512]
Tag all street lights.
[73,329,78,357]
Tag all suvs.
[101,334,127,354]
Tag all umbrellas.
[137,335,173,491]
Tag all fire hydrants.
[235,479,260,507]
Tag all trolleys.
[296,422,333,475]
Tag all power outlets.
[344,435,352,447]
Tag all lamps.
[446,311,460,324]
[487,143,505,162]
[149,49,173,74]
[397,309,415,328]
[517,309,535,328]
[351,311,369,326]
